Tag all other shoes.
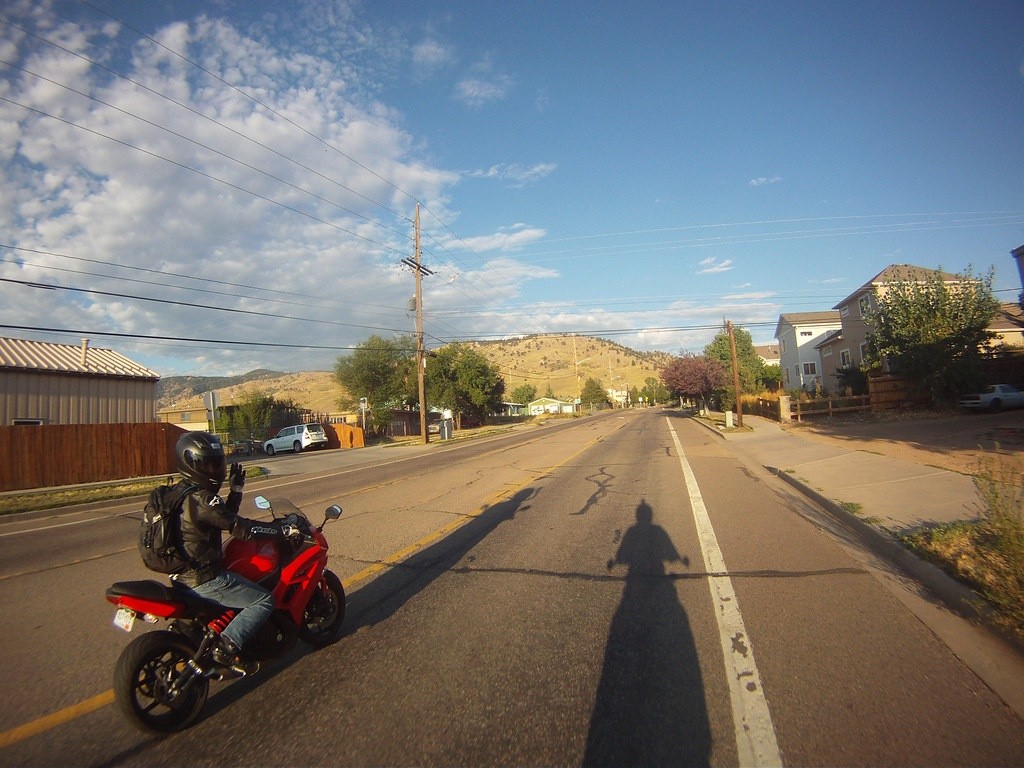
[213,645,258,675]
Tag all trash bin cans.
[438,418,452,439]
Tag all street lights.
[621,382,629,409]
[576,357,591,416]
[610,375,620,410]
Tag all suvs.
[263,422,328,456]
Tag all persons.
[168,432,308,677]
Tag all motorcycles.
[104,494,347,736]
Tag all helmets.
[176,432,223,492]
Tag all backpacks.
[138,477,207,573]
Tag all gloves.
[228,462,246,494]
[282,523,301,539]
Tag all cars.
[428,419,442,434]
[958,383,1024,414]
